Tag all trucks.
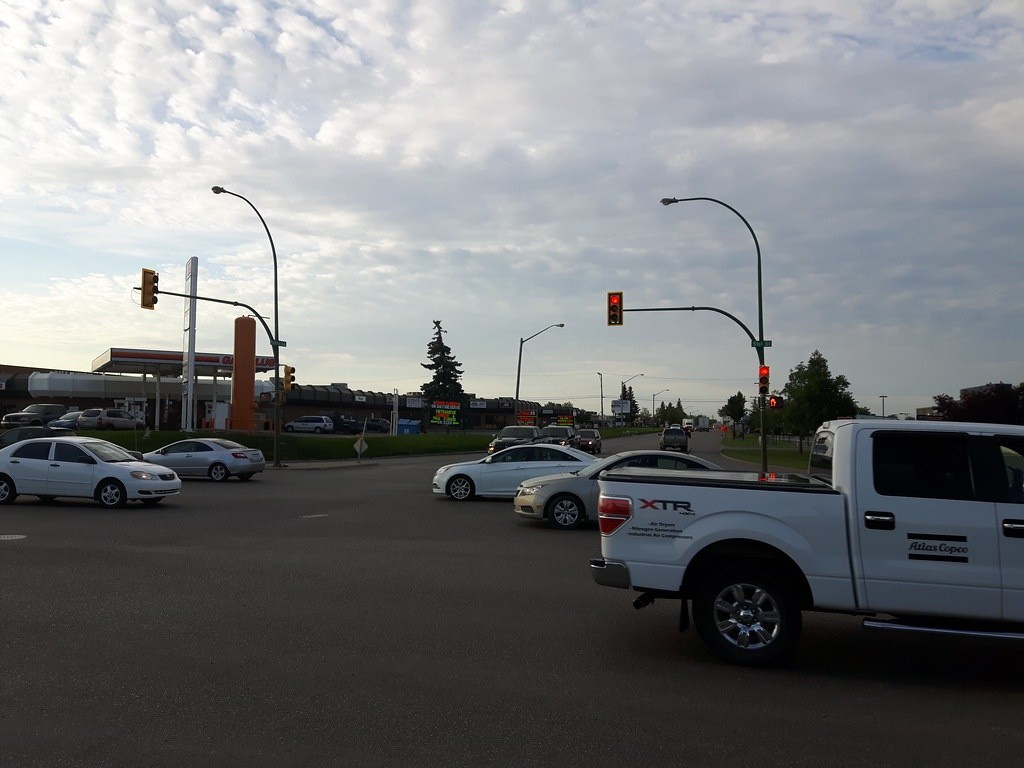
[682,419,694,430]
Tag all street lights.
[653,388,671,430]
[623,372,643,434]
[878,395,888,418]
[212,184,286,468]
[662,196,770,474]
[514,322,566,423]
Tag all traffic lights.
[772,397,786,409]
[139,267,160,309]
[758,364,772,395]
[607,291,625,325]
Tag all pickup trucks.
[589,416,1024,666]
[333,412,357,432]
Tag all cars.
[574,428,603,457]
[48,411,81,430]
[76,407,147,430]
[1,435,182,509]
[658,423,695,453]
[511,447,726,530]
[366,418,391,434]
[487,425,542,457]
[430,443,603,502]
[538,423,574,447]
[0,402,66,429]
[142,437,266,481]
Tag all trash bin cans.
[397,418,422,435]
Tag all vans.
[284,415,334,434]
